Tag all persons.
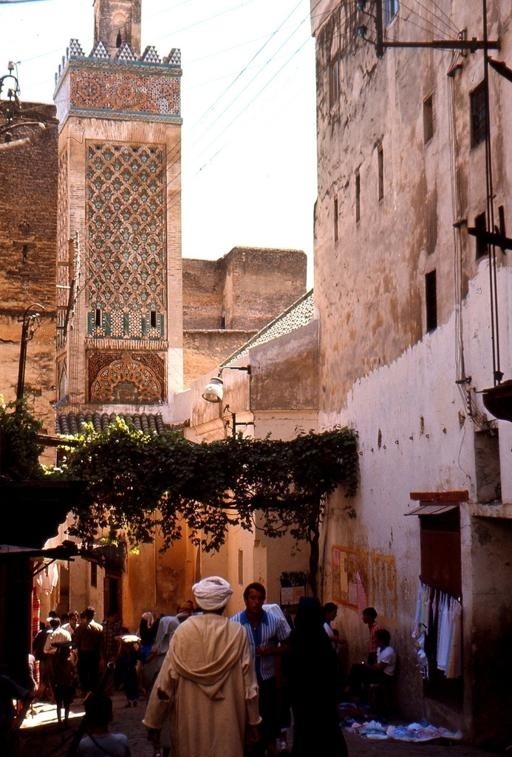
[324,602,348,643]
[362,607,384,668]
[229,582,291,695]
[272,595,349,756]
[351,629,397,692]
[1,600,194,757]
[143,575,262,756]
[332,629,345,659]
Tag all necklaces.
[251,623,259,631]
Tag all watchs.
[360,660,366,666]
